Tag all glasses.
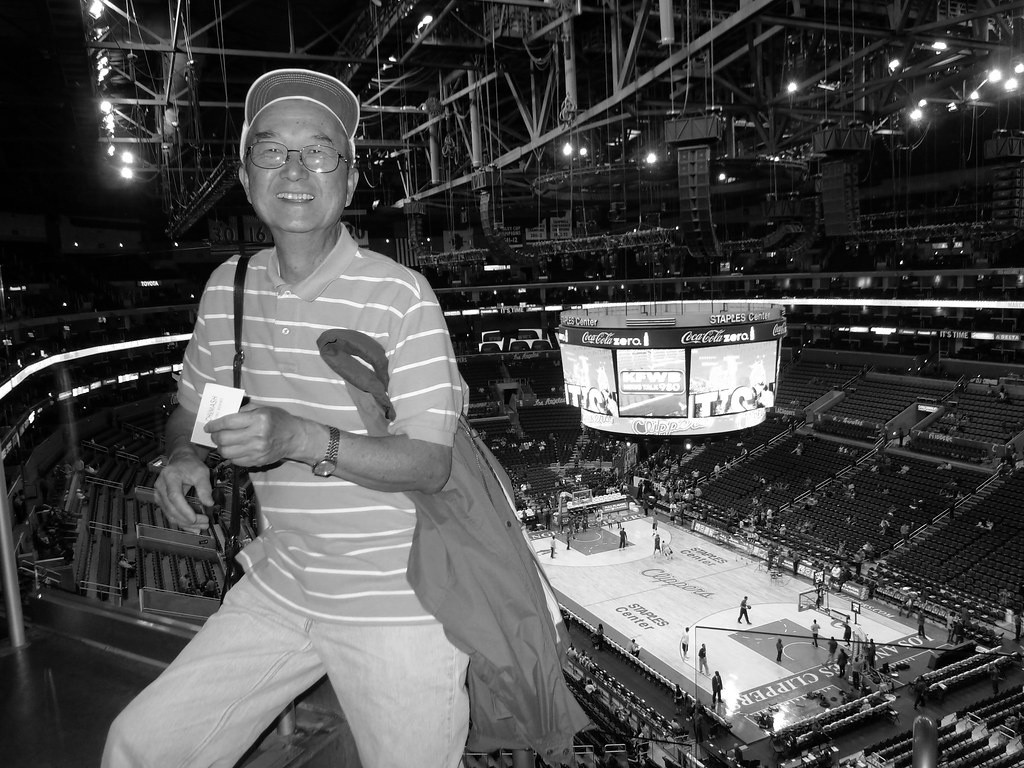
[241,141,351,174]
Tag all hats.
[235,67,359,169]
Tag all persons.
[0,357,1024,768]
[101,68,470,768]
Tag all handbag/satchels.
[211,460,260,556]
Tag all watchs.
[312,426,340,478]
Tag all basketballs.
[626,542,628,546]
[670,551,673,554]
[747,605,751,609]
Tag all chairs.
[28,398,1024,768]
